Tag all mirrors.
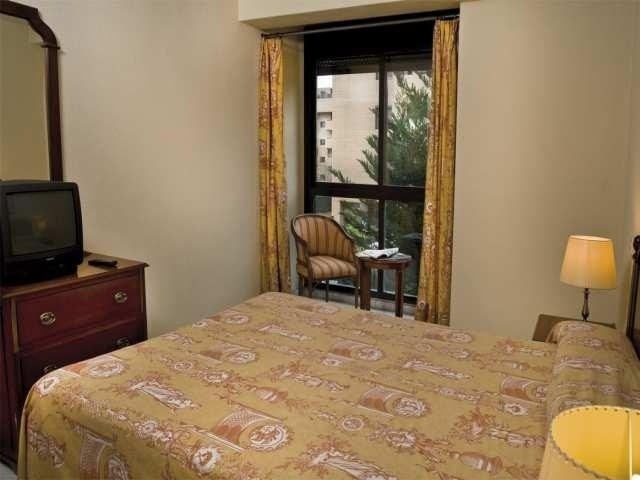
[0,0,65,183]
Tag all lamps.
[536,406,640,480]
[558,234,617,320]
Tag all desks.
[354,249,413,319]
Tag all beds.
[17,234,638,479]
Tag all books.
[355,247,400,259]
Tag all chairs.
[290,212,359,308]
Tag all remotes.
[88,259,118,267]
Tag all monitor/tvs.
[0,180,83,286]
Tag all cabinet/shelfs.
[0,248,148,473]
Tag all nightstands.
[533,313,618,345]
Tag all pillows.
[545,381,639,447]
[552,321,637,387]
